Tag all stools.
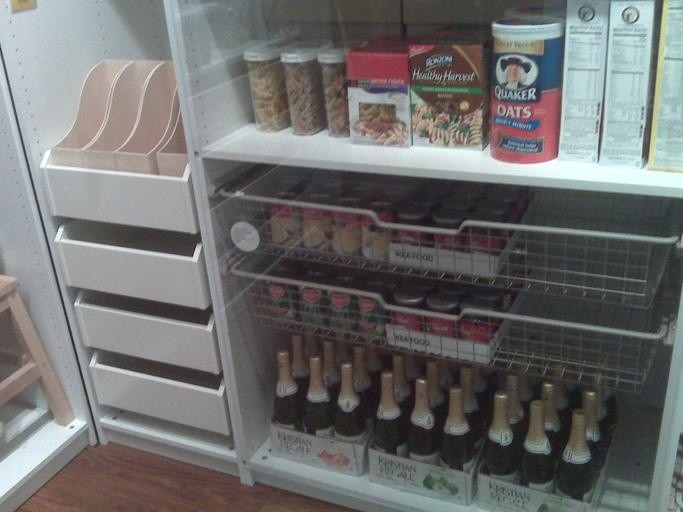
[1,267,78,429]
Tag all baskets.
[214,164,681,312]
[231,249,669,393]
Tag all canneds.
[489,13,566,163]
[268,172,529,344]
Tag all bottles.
[487,366,618,501]
[374,353,493,473]
[269,333,384,444]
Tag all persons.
[500,57,533,90]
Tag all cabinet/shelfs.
[1,1,248,487]
[168,0,682,512]
